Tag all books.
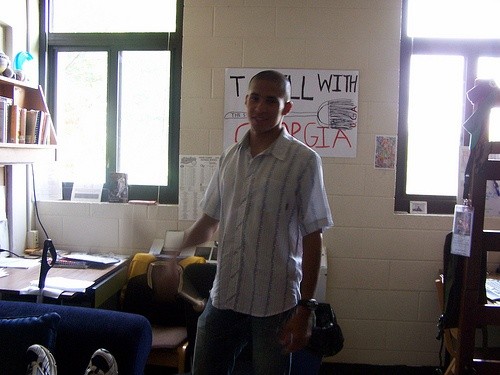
[0,95,51,145]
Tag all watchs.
[298,299,317,310]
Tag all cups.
[62,182,73,200]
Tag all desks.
[0,254,134,309]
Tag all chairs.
[122,273,188,375]
[435,269,500,375]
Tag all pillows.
[0,312,61,375]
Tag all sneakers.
[26,344,57,375]
[85,347,118,375]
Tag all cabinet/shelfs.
[0,76,59,164]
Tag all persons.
[169,70,334,375]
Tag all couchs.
[0,300,153,375]
